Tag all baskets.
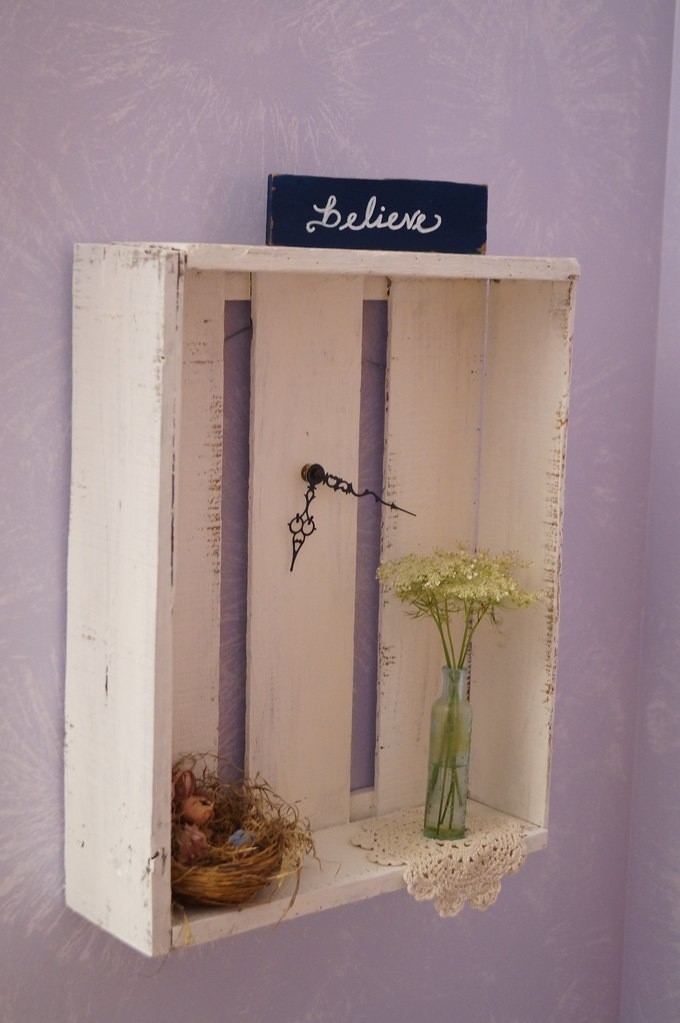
[171,833,284,905]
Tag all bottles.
[422,666,475,837]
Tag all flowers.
[373,547,547,669]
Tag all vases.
[423,666,474,841]
